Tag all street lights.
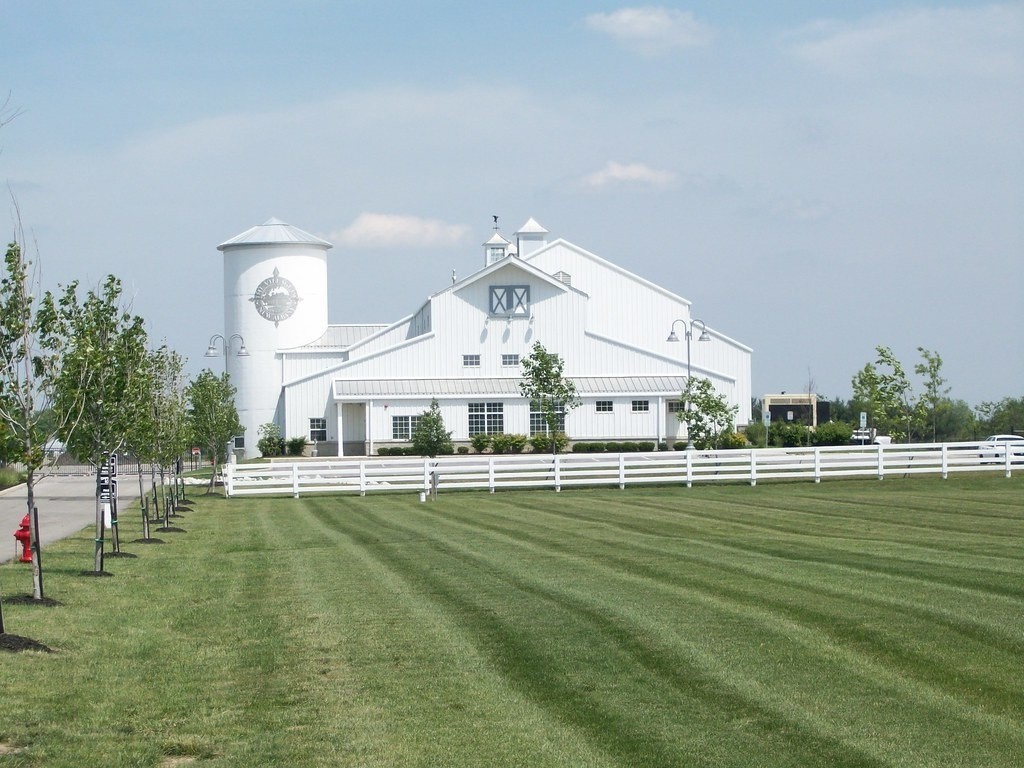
[204,332,250,378]
[666,318,711,452]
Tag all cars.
[978,435,1023,466]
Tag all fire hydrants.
[13,513,30,563]
